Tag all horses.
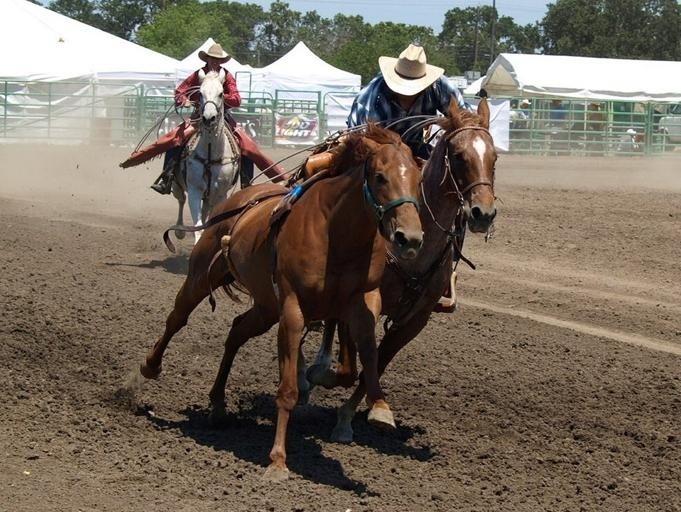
[171,67,244,247]
[136,119,427,483]
[295,92,500,446]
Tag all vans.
[657,102,681,143]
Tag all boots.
[151,169,174,195]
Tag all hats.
[197,43,231,64]
[378,43,445,96]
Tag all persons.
[150,43,254,194]
[510,99,639,151]
[346,43,470,160]
